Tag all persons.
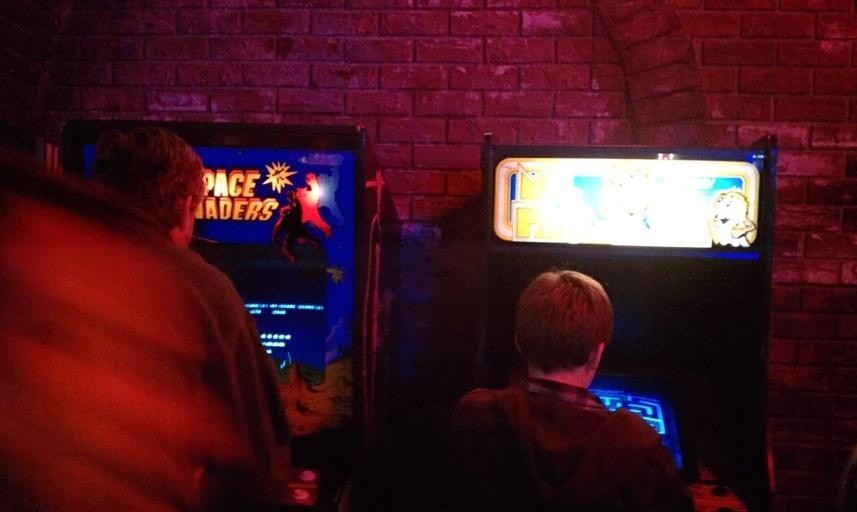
[273,171,331,259]
[443,267,694,511]
[0,127,294,511]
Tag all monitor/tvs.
[63,120,357,386]
[591,381,685,471]
